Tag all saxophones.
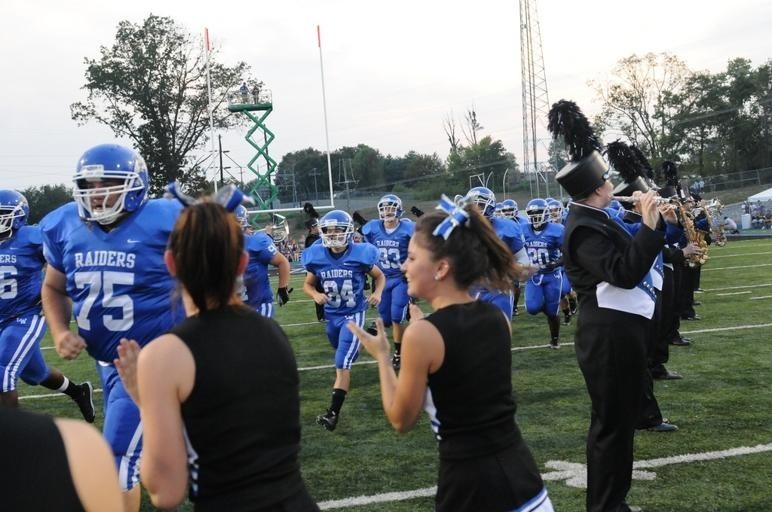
[666,200,708,266]
[705,202,727,246]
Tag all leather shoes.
[647,423,679,432]
[656,335,694,379]
[683,288,706,320]
[662,418,669,423]
[627,505,643,512]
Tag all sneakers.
[316,409,338,431]
[569,298,577,314]
[368,322,378,335]
[74,381,97,422]
[392,351,401,371]
[512,306,519,316]
[551,336,560,349]
[565,310,572,325]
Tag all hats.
[614,176,661,209]
[647,179,663,192]
[678,187,702,203]
[304,218,318,229]
[555,150,621,196]
[658,187,682,201]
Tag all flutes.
[610,195,662,205]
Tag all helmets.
[0,190,29,241]
[466,187,518,220]
[377,194,405,222]
[318,210,355,249]
[71,144,149,228]
[526,198,572,231]
[234,204,252,233]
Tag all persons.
[238,80,259,101]
[0,141,772,512]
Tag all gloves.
[276,286,293,307]
